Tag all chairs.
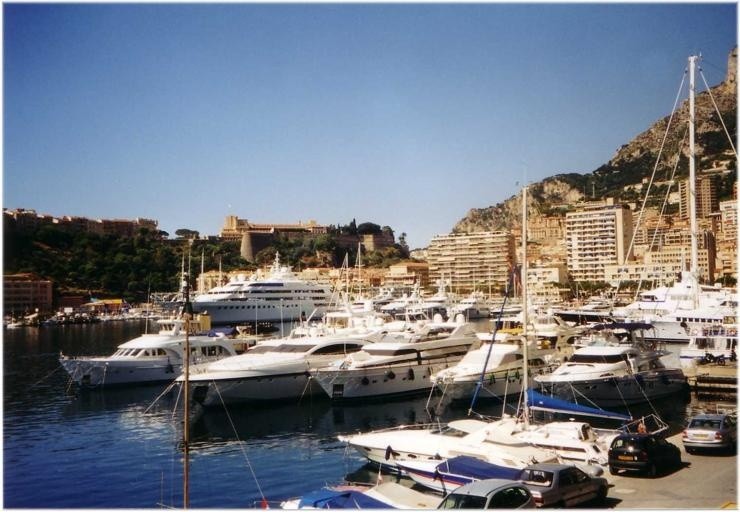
[695,420,721,428]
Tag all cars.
[514,463,609,508]
[609,434,681,479]
[436,478,537,509]
[683,415,737,456]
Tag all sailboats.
[154,50,738,355]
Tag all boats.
[531,346,688,409]
[429,342,562,400]
[280,409,609,509]
[57,272,239,385]
[305,319,521,400]
